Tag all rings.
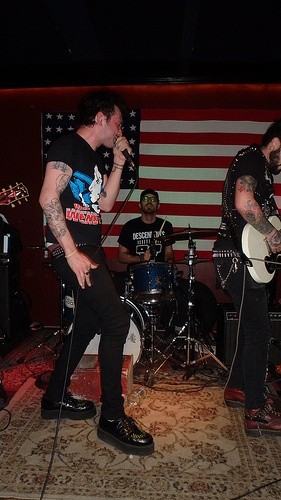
[85,272,90,275]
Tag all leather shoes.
[223,384,281,410]
[244,402,281,438]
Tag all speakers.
[0,262,22,340]
[220,303,281,368]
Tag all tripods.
[144,235,229,387]
[17,275,67,364]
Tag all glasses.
[141,196,157,202]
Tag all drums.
[126,261,178,305]
[67,296,147,369]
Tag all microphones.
[269,338,281,347]
[115,141,137,171]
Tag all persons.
[38,88,154,455]
[213,118,281,437]
[117,188,180,370]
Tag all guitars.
[240,215,281,284]
[0,182,30,208]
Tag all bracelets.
[65,249,78,257]
[113,162,124,169]
[140,255,144,262]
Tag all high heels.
[40,392,96,419]
[97,416,155,455]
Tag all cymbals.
[155,229,219,242]
[168,259,212,264]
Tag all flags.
[40,109,281,264]
